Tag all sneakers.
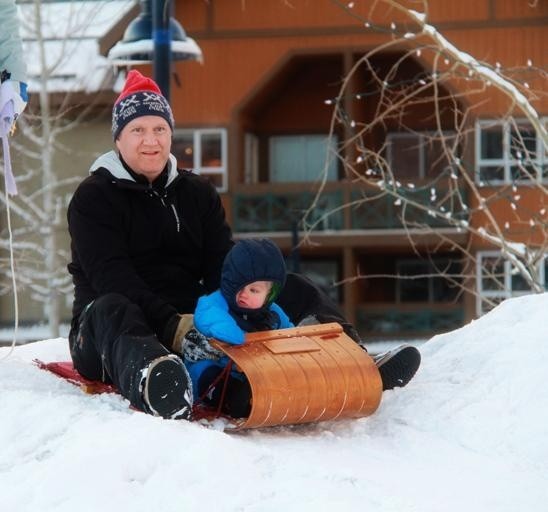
[371,343,421,390]
[146,355,194,424]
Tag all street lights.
[106,2,203,104]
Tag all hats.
[109,68,176,142]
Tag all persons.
[63,70,423,420]
[182,236,296,420]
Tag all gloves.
[174,313,224,365]
